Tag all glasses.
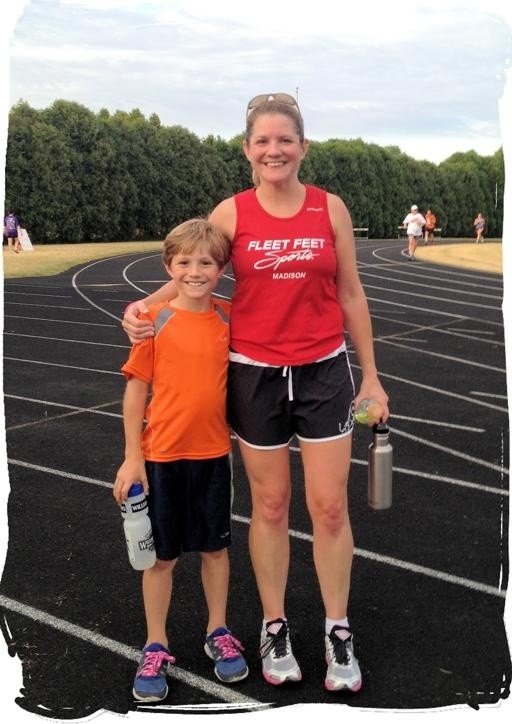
[247,93,302,128]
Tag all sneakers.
[260,618,302,686]
[325,625,362,692]
[132,642,176,702]
[204,626,249,682]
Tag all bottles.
[365,423,394,511]
[352,399,384,428]
[116,481,158,573]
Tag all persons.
[424,209,436,245]
[119,93,391,693]
[402,203,426,261]
[5,209,21,253]
[111,214,249,703]
[474,213,485,243]
